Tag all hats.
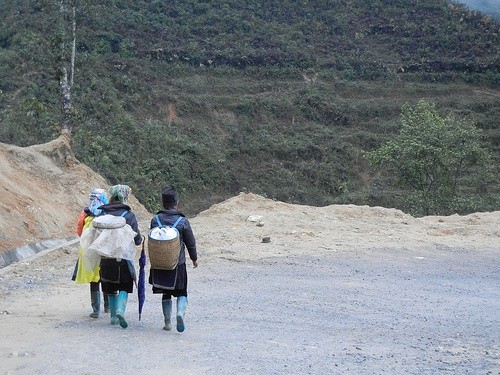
[162,191,178,204]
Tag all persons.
[72,189,112,318]
[148,187,198,333]
[91,184,142,328]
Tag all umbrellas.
[137,234,146,321]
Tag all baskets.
[148,226,180,270]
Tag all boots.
[162,299,172,331]
[109,294,118,325]
[116,291,128,328]
[90,291,100,317]
[177,296,188,332]
[103,291,110,312]
